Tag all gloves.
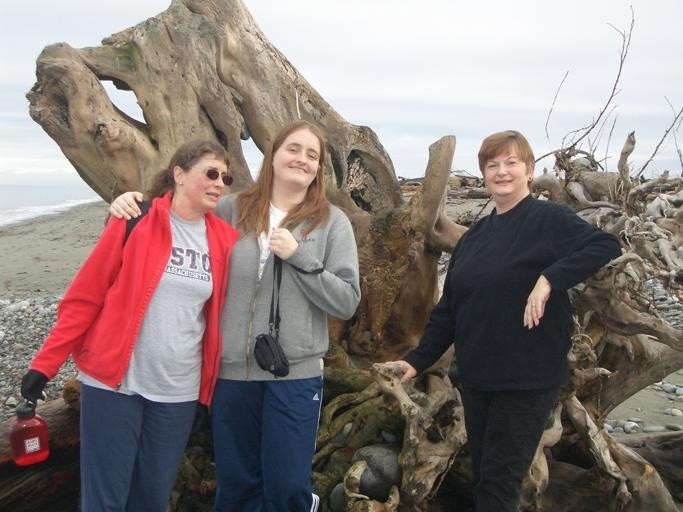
[19,370,47,403]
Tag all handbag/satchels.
[252,332,291,378]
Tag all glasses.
[202,168,235,187]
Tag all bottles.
[10,403,50,467]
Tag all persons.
[385,130,622,512]
[108,122,360,510]
[22,140,241,510]
